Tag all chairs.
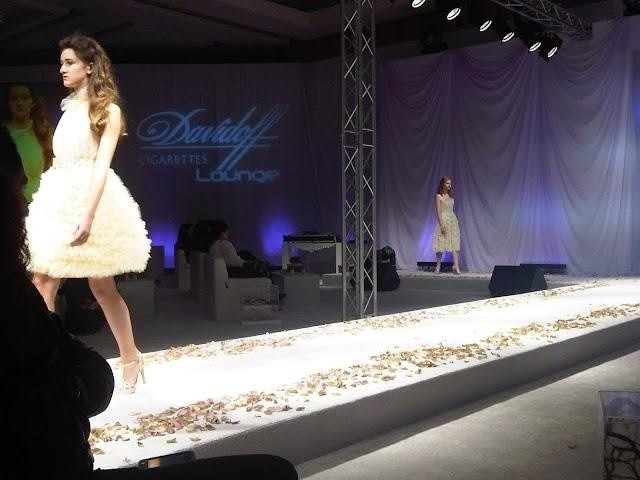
[175,249,191,291]
[189,250,207,304]
[203,254,272,322]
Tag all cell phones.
[138,450,196,471]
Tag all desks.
[281,241,342,274]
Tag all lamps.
[440,1,462,21]
[540,37,558,59]
[520,28,542,52]
[493,15,515,43]
[471,9,492,32]
[409,0,426,8]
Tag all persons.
[0,128,299,480]
[433,177,461,274]
[178,219,288,301]
[0,81,54,204]
[22,33,152,394]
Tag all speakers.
[487,265,548,297]
[351,263,401,291]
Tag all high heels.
[433,266,441,274]
[452,265,462,274]
[114,350,146,396]
